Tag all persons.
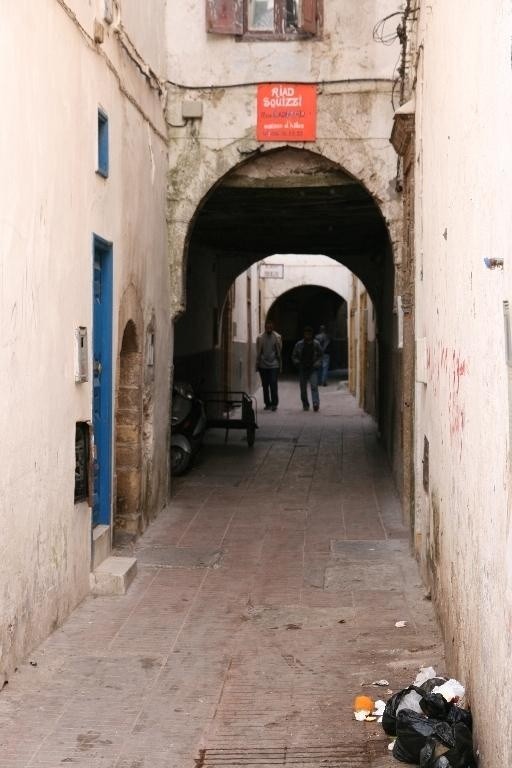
[254,319,283,411]
[315,324,333,386]
[291,325,323,412]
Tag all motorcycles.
[170,370,207,477]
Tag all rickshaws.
[199,391,260,447]
